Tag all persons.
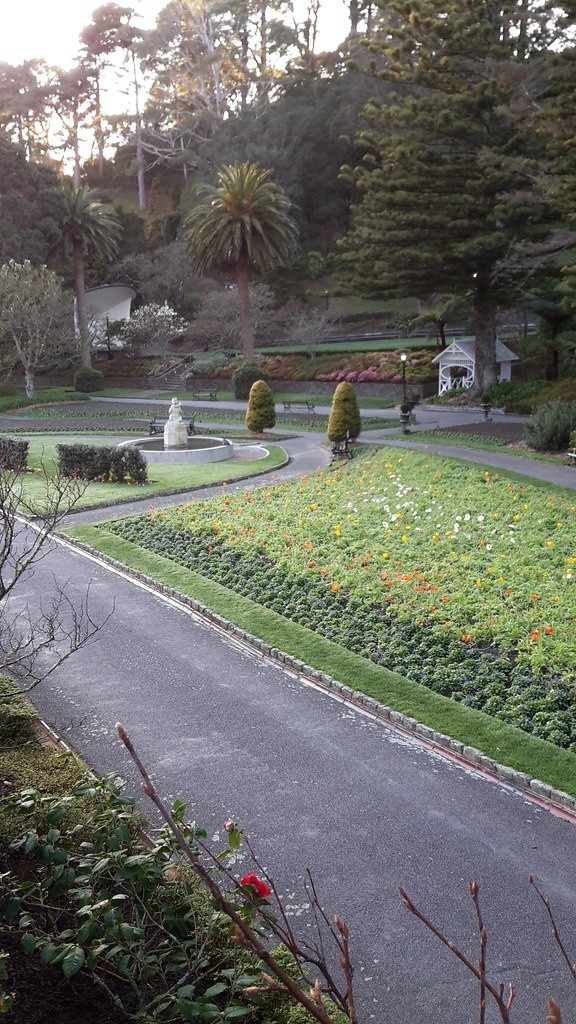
[169,398,183,422]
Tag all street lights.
[401,352,407,402]
[106,312,114,359]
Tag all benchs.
[331,429,352,459]
[149,413,195,435]
[282,400,315,413]
[192,388,217,400]
[567,447,576,465]
